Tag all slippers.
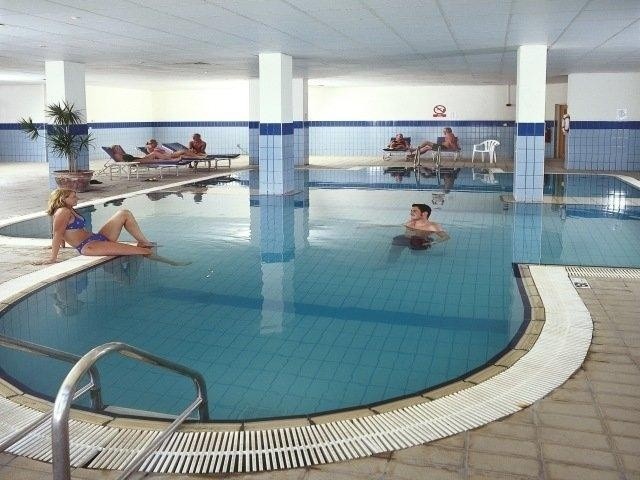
[152,177,159,181]
[145,178,154,182]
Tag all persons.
[390,172,407,182]
[113,192,185,206]
[112,144,182,165]
[416,167,456,206]
[52,255,148,316]
[144,139,207,160]
[407,126,457,157]
[35,186,195,272]
[187,132,207,170]
[388,133,411,151]
[356,202,452,270]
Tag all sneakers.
[90,179,104,184]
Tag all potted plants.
[18,101,96,192]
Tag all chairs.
[471,140,500,163]
[381,166,499,185]
[382,136,412,161]
[433,136,462,163]
[97,141,241,181]
